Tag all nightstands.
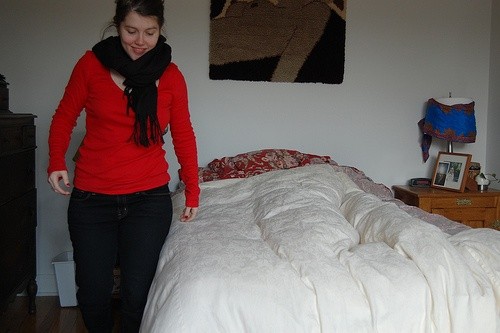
[391,184,500,229]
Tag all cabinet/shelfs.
[0,73,38,315]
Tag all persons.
[446,166,455,182]
[45,0,201,333]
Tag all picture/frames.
[430,151,472,193]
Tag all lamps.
[417,91,477,163]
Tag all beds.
[138,149,500,333]
